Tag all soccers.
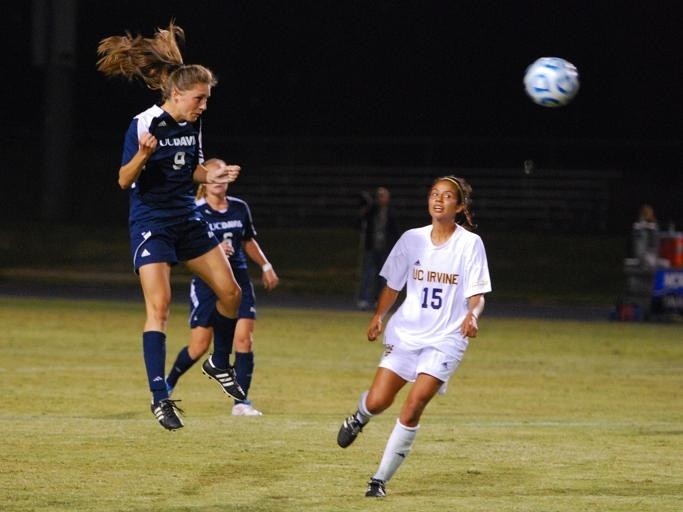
[523,56,580,108]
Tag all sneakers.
[201,353,246,400]
[337,407,369,448]
[232,402,262,416]
[366,477,386,497]
[151,398,185,430]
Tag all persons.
[161,153,280,418]
[354,185,409,309]
[94,16,247,433]
[331,175,493,499]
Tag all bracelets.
[259,262,274,272]
